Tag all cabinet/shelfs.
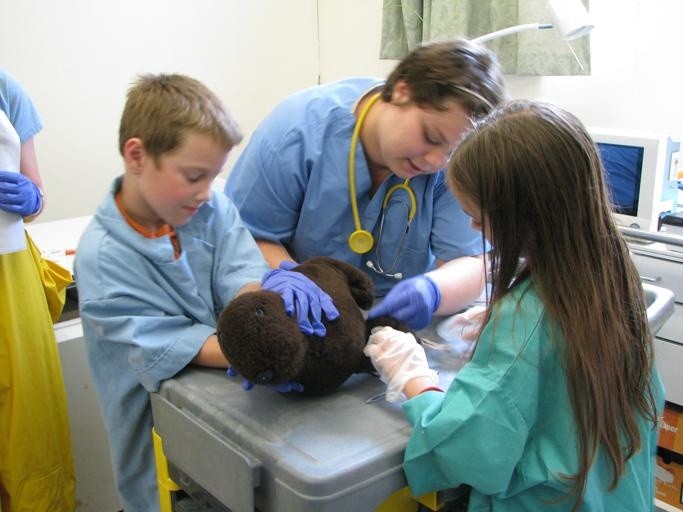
[625,231,683,407]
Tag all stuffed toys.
[219,255,421,400]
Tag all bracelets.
[419,387,445,395]
[28,189,45,221]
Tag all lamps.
[468,1,595,42]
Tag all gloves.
[367,274,440,330]
[259,269,339,339]
[0,171,41,216]
[227,366,305,394]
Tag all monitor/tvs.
[589,132,682,233]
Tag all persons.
[224,37,507,336]
[362,97,666,512]
[73,72,305,512]
[0,68,73,512]
